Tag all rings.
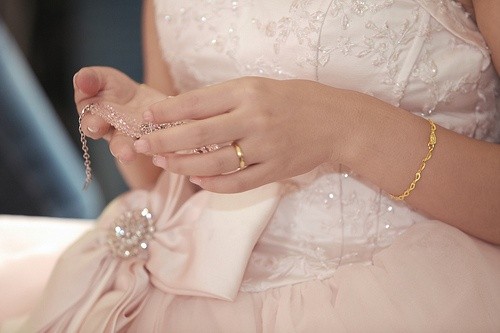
[231,143,246,170]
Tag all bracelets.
[389,119,436,201]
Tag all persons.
[13,0,500,331]
